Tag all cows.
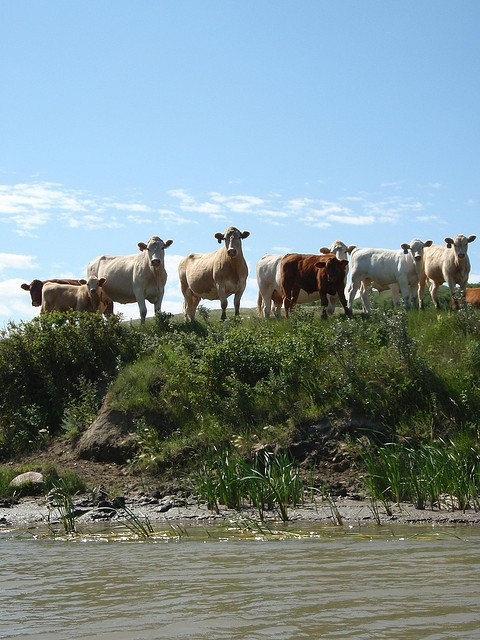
[255,240,358,319]
[279,252,350,321]
[20,278,114,319]
[418,234,477,311]
[178,226,250,325]
[347,239,433,314]
[86,235,174,327]
[40,274,107,316]
